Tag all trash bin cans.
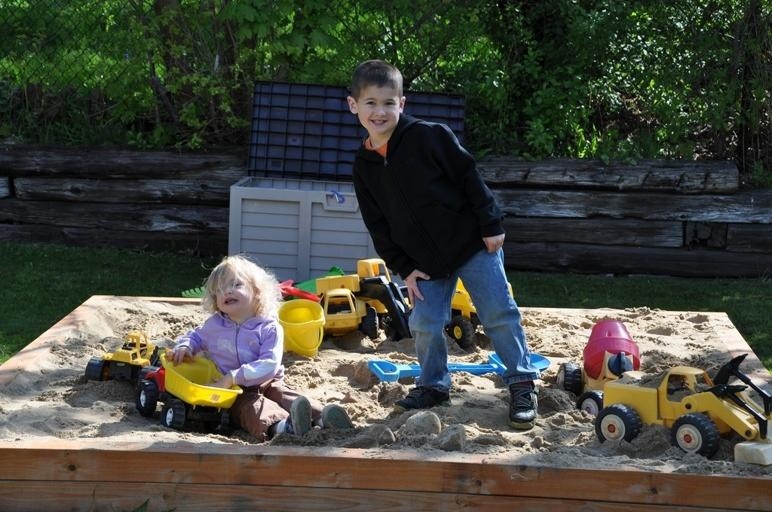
[228,80,467,284]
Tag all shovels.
[368,352,550,381]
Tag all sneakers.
[284,396,313,436]
[321,404,354,430]
[507,380,539,429]
[392,384,451,414]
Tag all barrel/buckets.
[278,299,326,356]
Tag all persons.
[347,58,540,430]
[167,254,353,442]
